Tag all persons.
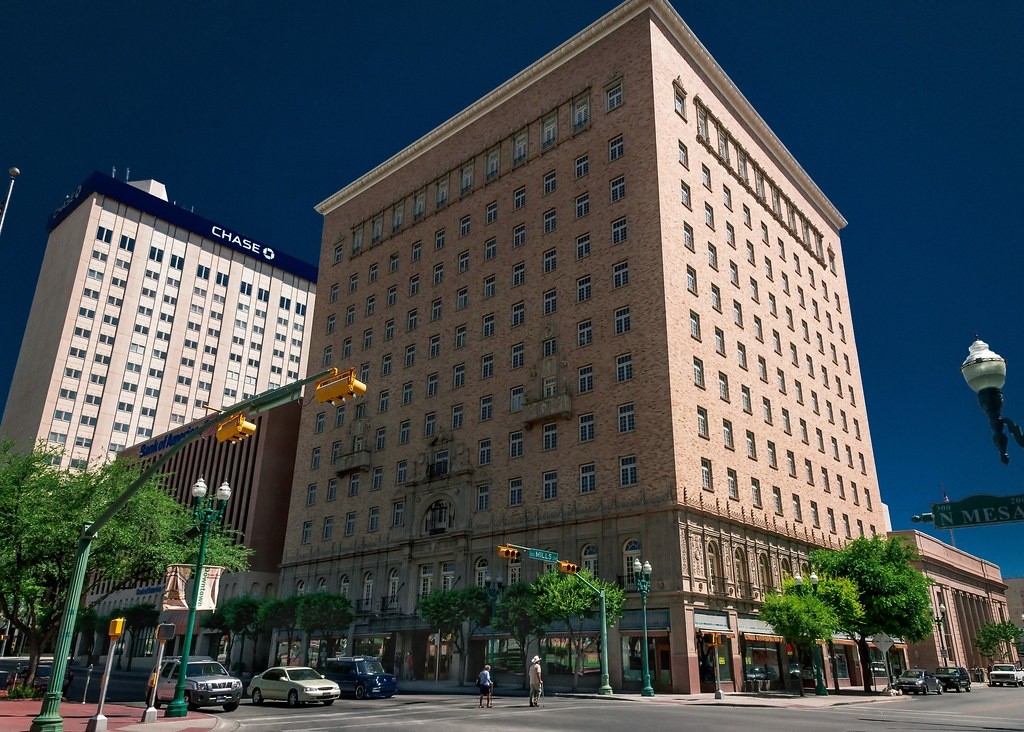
[987,664,992,685]
[407,652,414,681]
[529,655,543,707]
[476,665,493,708]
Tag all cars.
[895,668,944,695]
[745,665,777,682]
[6,664,71,699]
[246,665,341,708]
[932,666,971,693]
[870,661,887,677]
[789,663,825,679]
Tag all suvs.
[145,655,244,712]
[324,655,400,700]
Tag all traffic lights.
[497,545,521,560]
[558,561,580,574]
[314,375,367,407]
[216,411,256,445]
[703,633,715,645]
[156,623,177,642]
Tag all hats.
[531,655,542,664]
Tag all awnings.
[699,629,735,638]
[743,633,785,642]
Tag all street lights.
[633,557,655,697]
[927,603,948,666]
[163,473,233,719]
[795,571,829,695]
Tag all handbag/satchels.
[475,678,480,688]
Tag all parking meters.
[81,663,94,704]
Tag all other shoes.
[479,705,484,708]
[487,705,492,708]
[530,704,534,707]
[534,702,539,707]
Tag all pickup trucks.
[989,663,1024,687]
